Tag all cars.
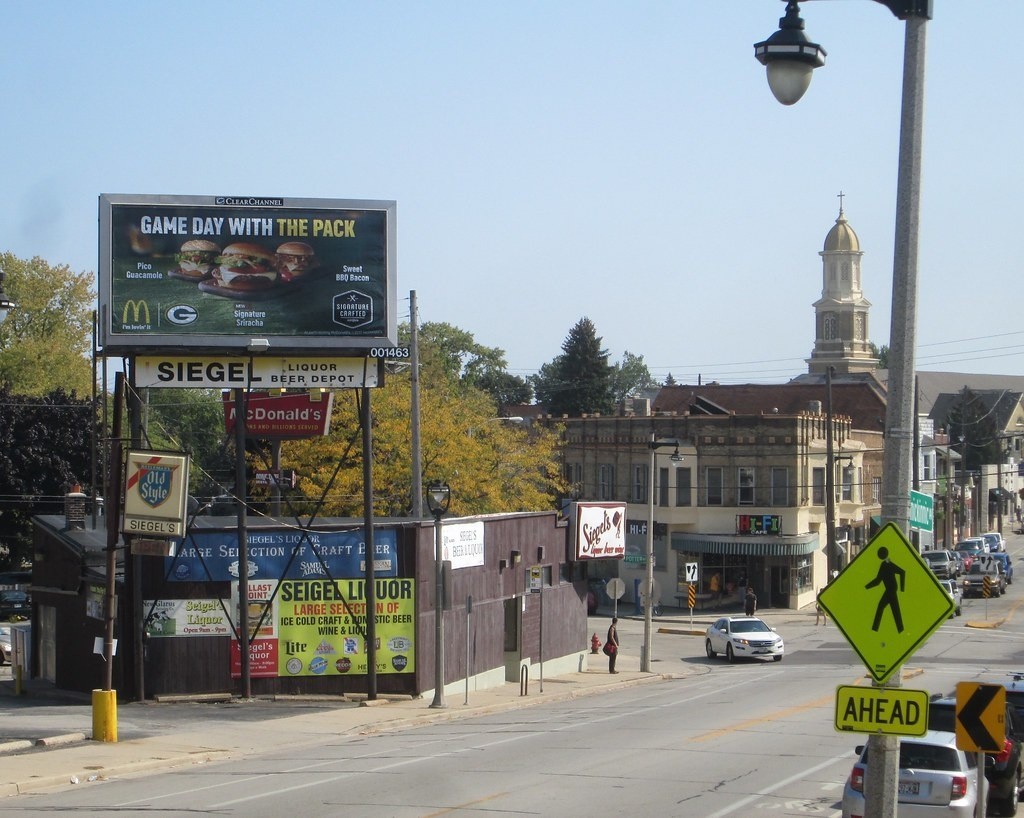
[915,668,1024,818]
[0,589,32,621]
[961,559,1008,598]
[704,615,784,664]
[0,623,14,665]
[841,727,996,818]
[937,578,963,620]
[972,552,1014,585]
[922,532,1006,581]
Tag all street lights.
[641,439,688,672]
[945,423,967,549]
[426,478,450,709]
[467,417,525,438]
[752,0,934,816]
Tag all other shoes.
[610,671,619,674]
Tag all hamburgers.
[174,239,317,292]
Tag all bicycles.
[639,600,665,616]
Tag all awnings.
[936,446,962,463]
[989,487,1013,501]
[671,531,821,556]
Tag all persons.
[607,617,620,674]
[710,571,721,610]
[743,588,757,616]
[1016,506,1022,522]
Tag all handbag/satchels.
[602,643,615,656]
